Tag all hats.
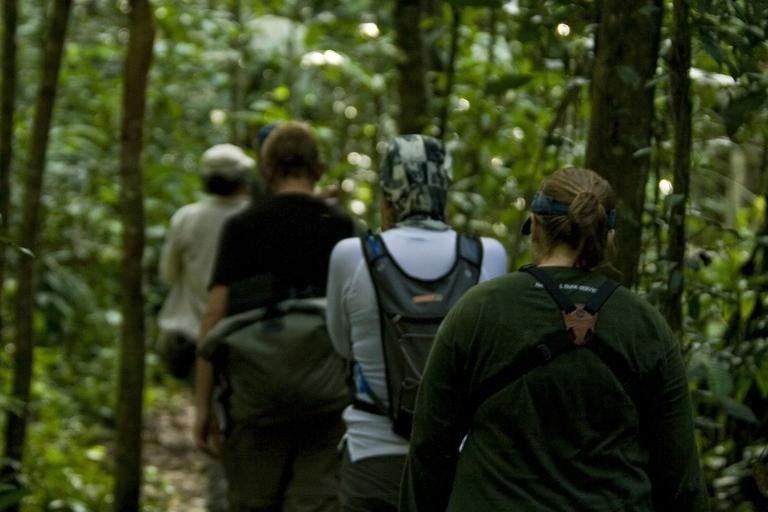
[520,190,617,235]
[200,143,255,179]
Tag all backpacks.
[354,228,482,438]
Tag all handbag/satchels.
[190,296,352,419]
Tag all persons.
[323,130,512,510]
[189,118,369,512]
[158,140,258,380]
[398,164,710,510]
